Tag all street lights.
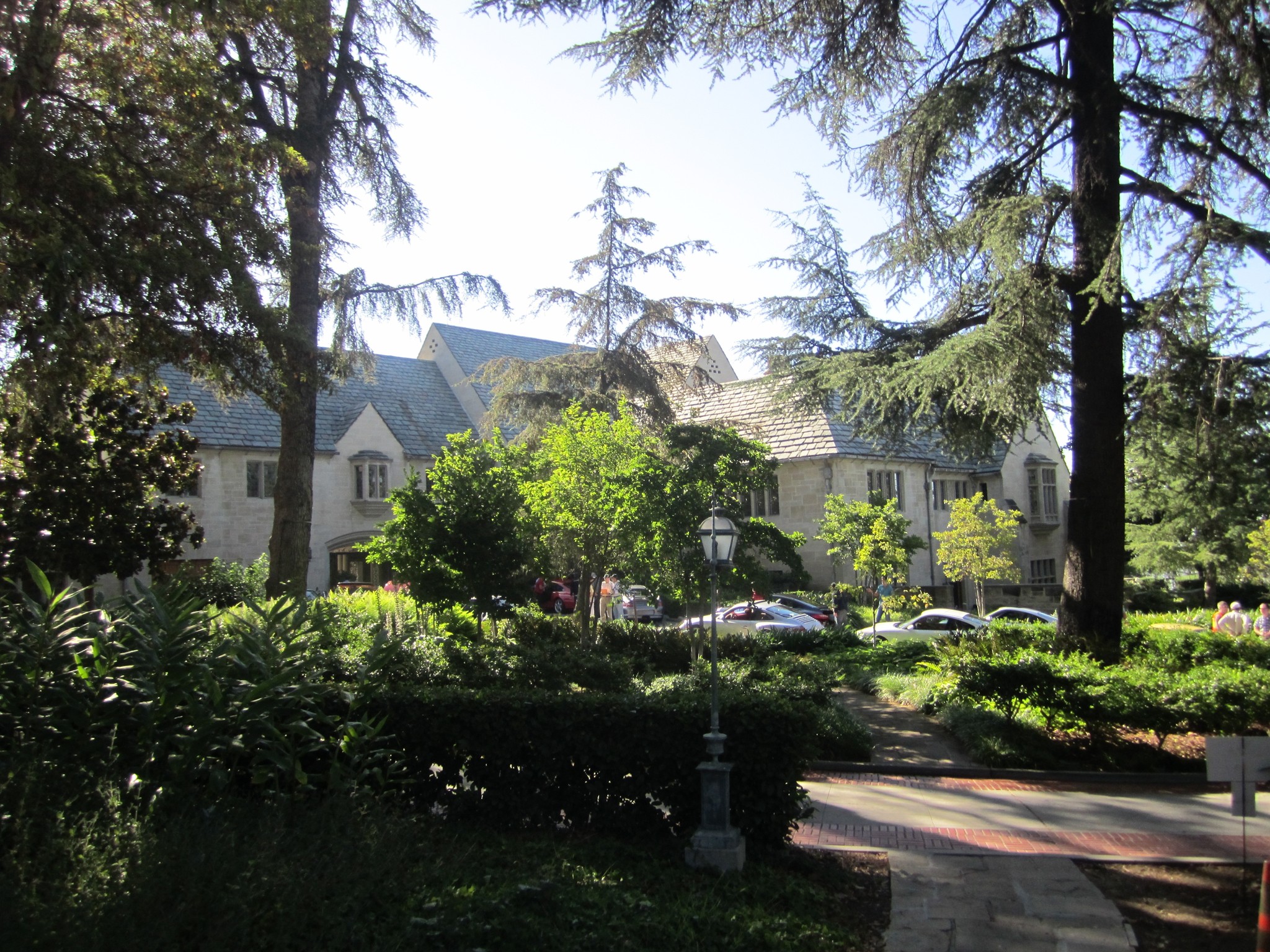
[683,487,746,876]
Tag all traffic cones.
[1256,860,1270,950]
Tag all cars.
[532,578,577,615]
[855,605,1058,646]
[678,593,834,645]
[574,584,663,622]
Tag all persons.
[570,575,580,603]
[1253,603,1270,640]
[610,574,625,619]
[1218,601,1250,637]
[599,574,614,622]
[589,572,597,617]
[830,582,848,625]
[866,574,893,623]
[752,588,764,600]
[1212,601,1229,632]
[534,576,547,606]
